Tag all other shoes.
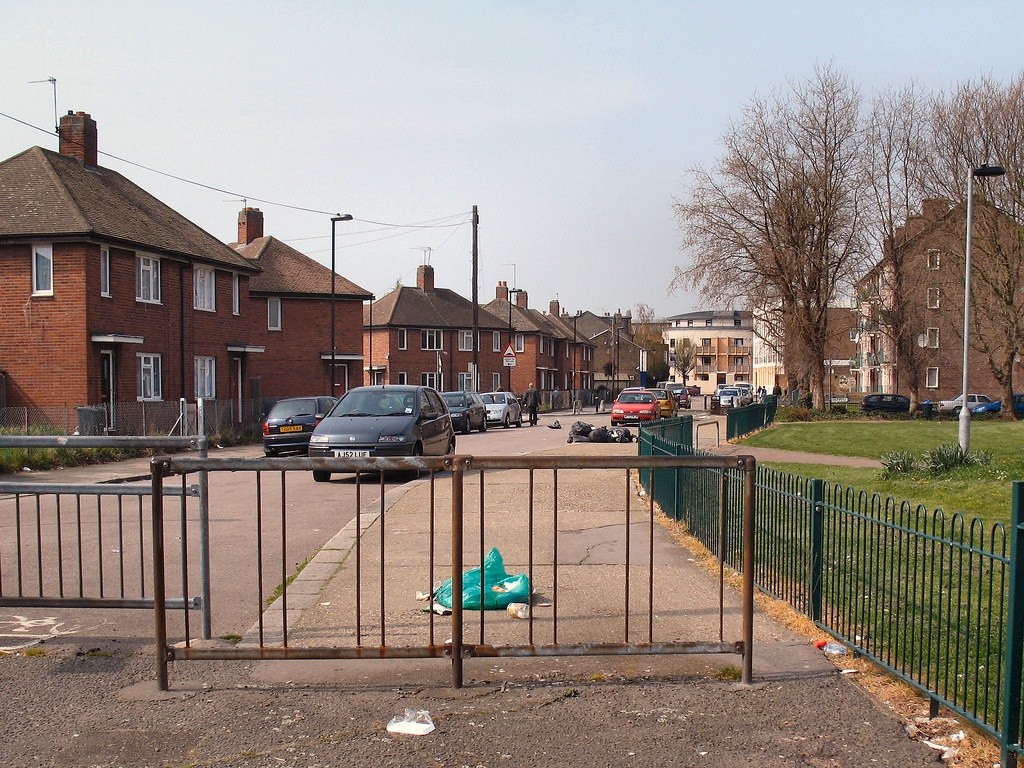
[534,422,537,425]
[530,423,533,426]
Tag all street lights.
[572,315,583,413]
[330,212,353,397]
[959,165,1007,455]
[617,326,625,396]
[507,289,522,393]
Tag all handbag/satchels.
[433,547,529,610]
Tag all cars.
[478,391,523,429]
[656,381,691,409]
[616,386,646,404]
[308,384,456,482]
[713,382,754,412]
[971,393,1024,419]
[938,393,994,417]
[610,390,660,428]
[861,393,923,416]
[637,387,680,418]
[262,395,339,457]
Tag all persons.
[520,383,541,426]
[552,386,562,412]
[496,386,504,392]
[758,386,766,403]
[773,383,782,399]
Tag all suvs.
[431,389,488,435]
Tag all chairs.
[403,395,414,413]
[643,397,651,402]
[627,397,635,401]
[364,397,382,412]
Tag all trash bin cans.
[711,396,720,415]
[75,406,105,437]
